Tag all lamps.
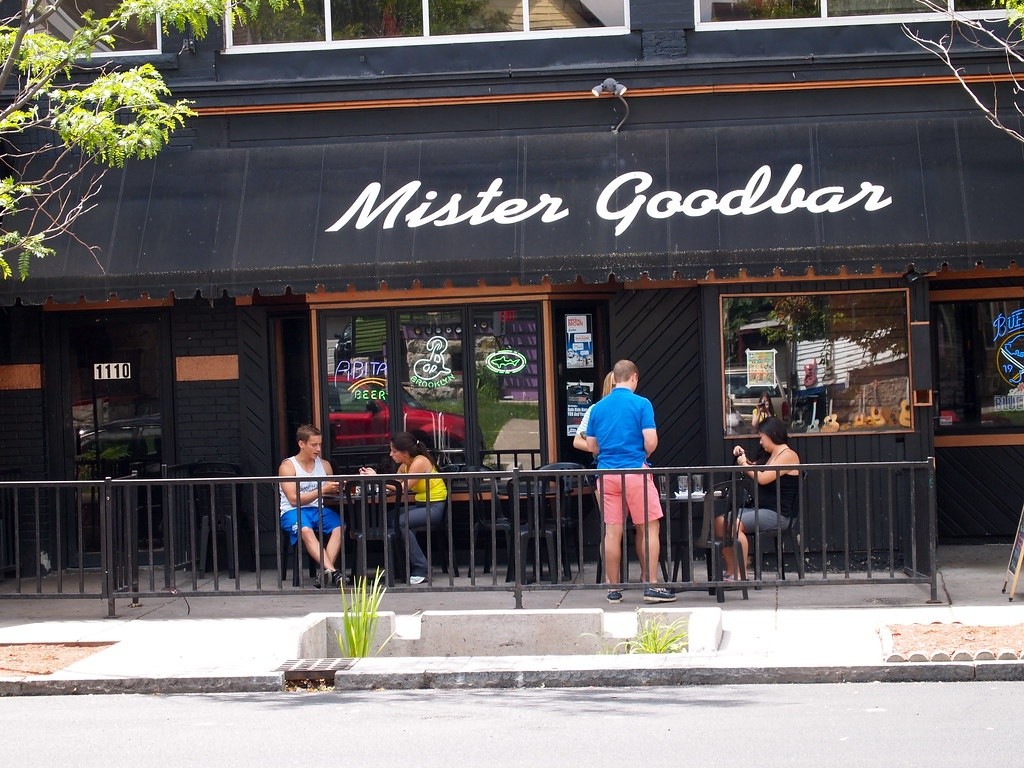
[902,271,920,284]
[591,77,631,136]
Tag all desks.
[323,484,597,581]
[660,490,723,582]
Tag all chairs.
[746,470,808,591]
[506,475,572,584]
[411,462,467,579]
[536,461,594,573]
[194,462,242,578]
[461,465,511,578]
[280,531,315,588]
[670,479,753,603]
[345,480,404,587]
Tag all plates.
[677,492,705,499]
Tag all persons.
[359,432,447,585]
[586,359,676,603]
[714,417,800,582]
[279,423,350,589]
[573,372,617,584]
[752,392,777,433]
[726,396,744,435]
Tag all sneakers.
[410,575,424,584]
[644,587,676,602]
[315,572,327,587]
[331,571,349,588]
[607,591,623,603]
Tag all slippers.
[724,573,750,583]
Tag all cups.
[659,476,667,495]
[692,474,704,493]
[677,475,688,494]
